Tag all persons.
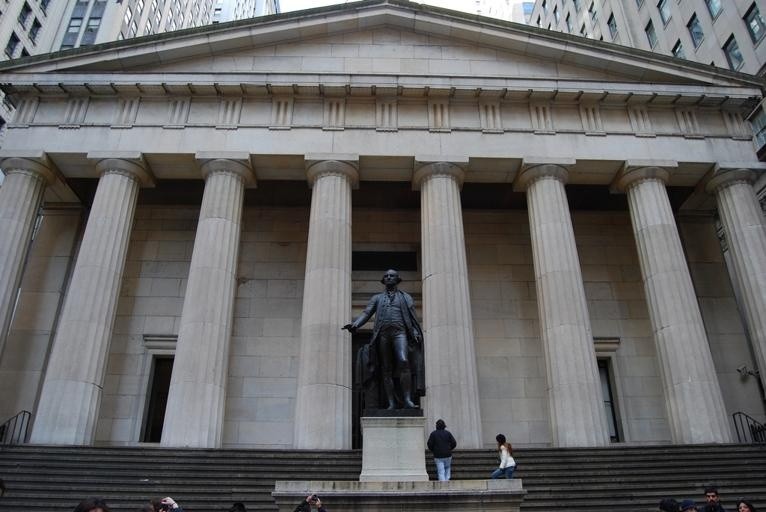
[699,488,729,512]
[428,419,457,480]
[736,499,757,512]
[142,495,184,511]
[341,270,424,411]
[229,502,246,512]
[74,495,111,512]
[680,499,701,511]
[660,498,681,511]
[491,433,517,479]
[293,494,328,512]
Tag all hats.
[680,500,697,510]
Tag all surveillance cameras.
[737,364,746,373]
